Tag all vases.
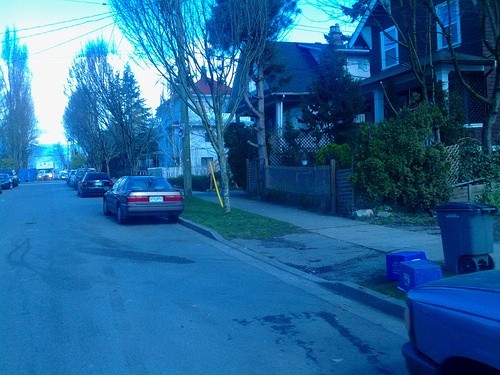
[302,160,309,165]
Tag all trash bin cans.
[434,201,498,274]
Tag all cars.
[78,172,113,197]
[37,168,96,191]
[401,269,500,375]
[103,175,184,224]
[0,169,19,195]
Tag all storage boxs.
[386,248,427,281]
[396,259,443,292]
